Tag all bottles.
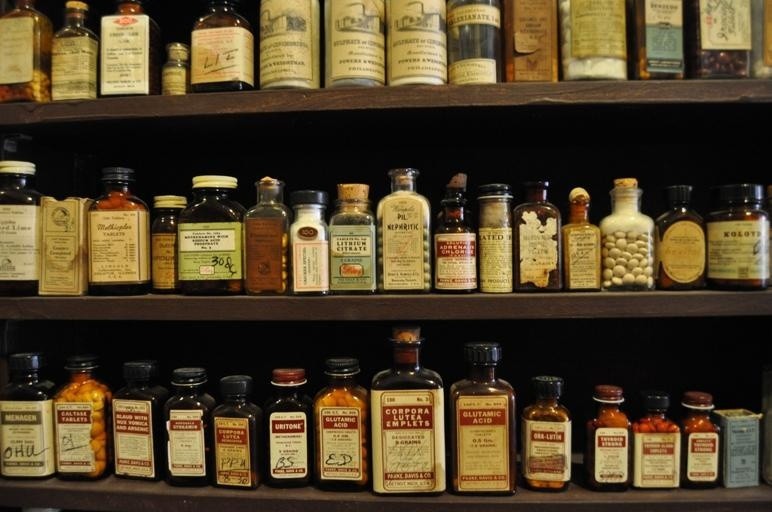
[3,0,772,102]
[0,325,764,494]
[0,155,770,288]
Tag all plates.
[0,76,771,512]
